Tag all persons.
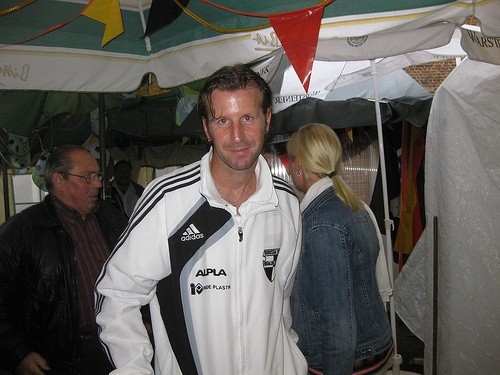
[286,124,395,375]
[0,143,131,375]
[94,62,309,375]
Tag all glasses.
[57,172,105,184]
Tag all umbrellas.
[0,0,500,215]
[250,22,500,370]
